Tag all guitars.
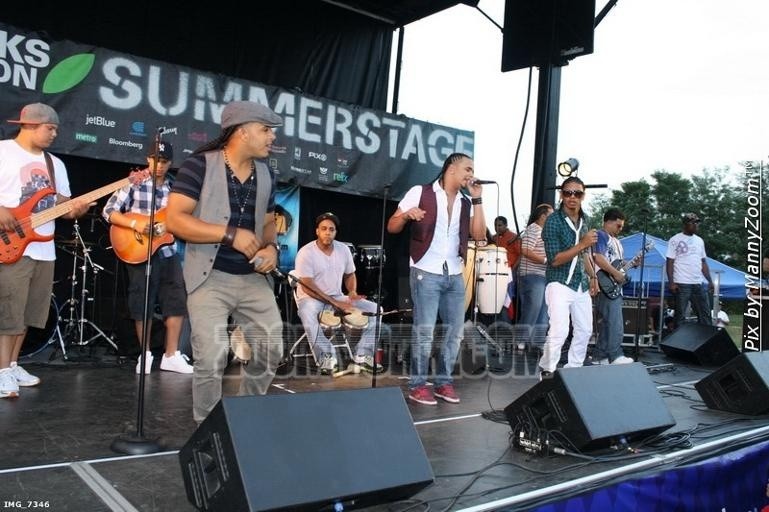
[596,240,656,300]
[0,168,153,264]
[110,206,174,264]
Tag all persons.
[666,213,715,330]
[294,212,384,374]
[387,153,487,405]
[0,103,98,397]
[591,209,643,365]
[166,101,285,427]
[648,297,730,346]
[370,249,383,267]
[102,141,193,375]
[518,204,555,347]
[492,216,522,324]
[538,177,601,380]
[361,250,369,266]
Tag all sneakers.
[321,354,338,374]
[409,385,437,405]
[611,356,633,364]
[10,361,40,386]
[351,354,384,373]
[433,384,460,403]
[591,357,609,365]
[136,350,153,374]
[160,351,193,374]
[0,369,20,398]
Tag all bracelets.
[348,291,357,296]
[590,275,597,279]
[472,197,481,204]
[222,225,237,248]
[129,219,136,228]
[544,256,547,265]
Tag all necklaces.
[223,145,255,228]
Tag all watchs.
[264,241,280,251]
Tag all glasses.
[562,190,585,199]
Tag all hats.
[682,212,701,225]
[149,141,173,161]
[222,101,283,129]
[8,103,59,125]
[315,212,340,227]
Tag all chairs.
[277,269,359,376]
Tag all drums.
[361,248,386,273]
[342,309,369,347]
[357,245,382,262]
[51,279,71,317]
[17,294,59,359]
[226,325,251,364]
[475,247,508,314]
[341,242,358,263]
[318,309,342,340]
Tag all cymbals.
[82,206,102,220]
[55,239,98,250]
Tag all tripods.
[633,215,677,373]
[463,248,504,365]
[27,217,124,366]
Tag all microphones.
[506,233,521,246]
[469,178,497,186]
[591,225,597,256]
[641,207,651,221]
[270,266,288,281]
[361,311,386,316]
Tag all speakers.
[504,363,676,452]
[178,387,434,511]
[741,286,764,355]
[659,322,741,367]
[694,350,769,415]
